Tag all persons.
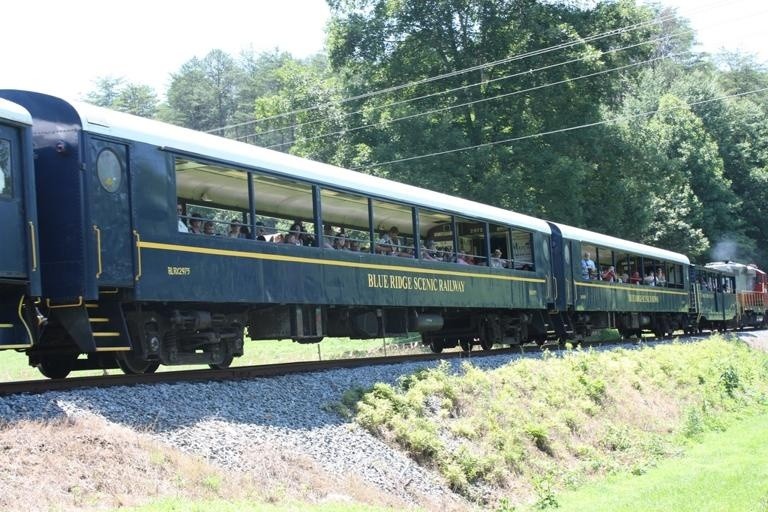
[376,227,415,259]
[176,198,266,241]
[420,242,453,262]
[698,277,730,294]
[457,249,474,264]
[273,224,316,247]
[579,251,668,285]
[324,226,367,252]
[490,250,511,268]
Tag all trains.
[0,90,768,387]
